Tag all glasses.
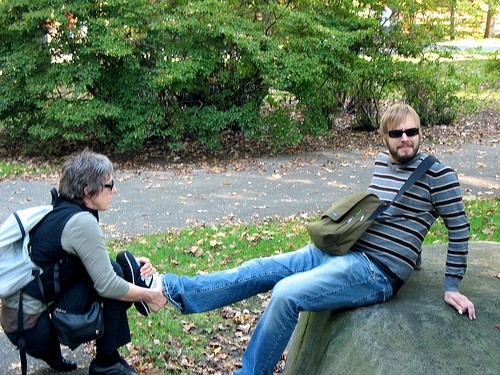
[102,180,114,191]
[389,128,420,139]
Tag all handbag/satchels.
[305,189,387,256]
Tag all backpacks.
[0,190,84,299]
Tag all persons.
[0,151,169,375]
[116,104,476,375]
[380,2,392,48]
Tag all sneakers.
[89,358,139,374]
[43,346,78,371]
[117,250,153,316]
